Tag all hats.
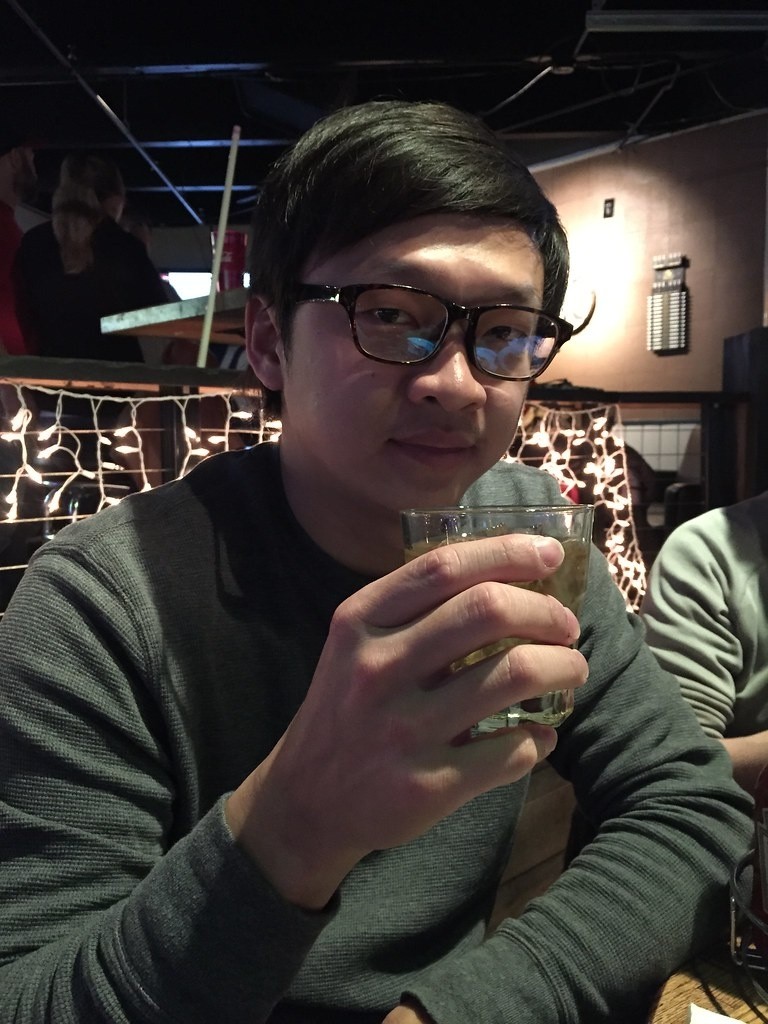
[0,120,26,158]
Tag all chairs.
[0,199,115,566]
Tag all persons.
[0,100,767,1024]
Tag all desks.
[99,287,249,346]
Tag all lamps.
[582,0,768,32]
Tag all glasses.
[292,281,573,381]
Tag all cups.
[403,501,595,731]
